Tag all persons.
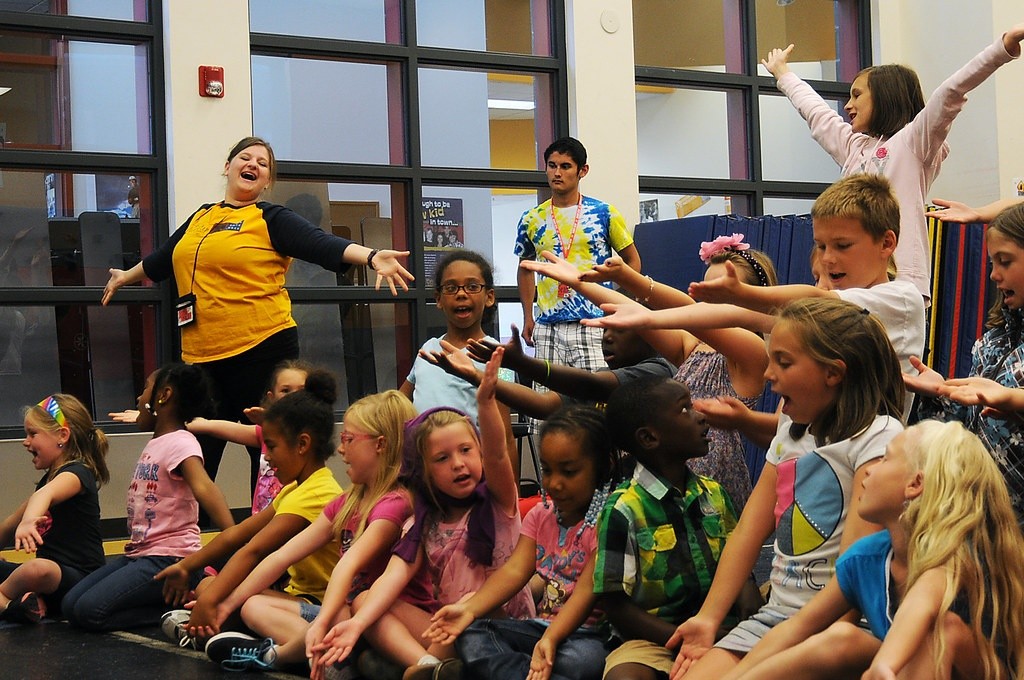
[100,136,414,531]
[0,393,108,623]
[108,173,1024,680]
[514,136,642,443]
[760,25,1024,424]
[61,362,235,630]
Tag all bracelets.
[636,275,654,302]
[367,248,379,269]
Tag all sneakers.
[205,631,276,672]
[308,649,356,680]
[158,610,200,652]
[6,591,48,625]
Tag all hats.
[129,176,136,181]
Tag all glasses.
[439,283,485,295]
[337,431,376,441]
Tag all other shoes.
[358,650,461,680]
